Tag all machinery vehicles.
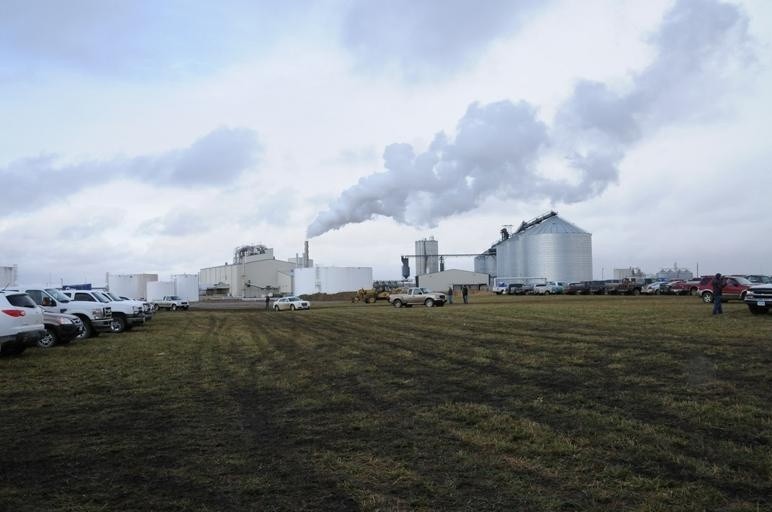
[357,287,401,303]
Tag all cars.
[492,275,772,314]
[0,288,156,348]
[273,297,311,312]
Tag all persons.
[448,287,453,304]
[711,273,728,315]
[265,295,270,312]
[461,285,468,304]
[42,297,51,306]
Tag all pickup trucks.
[153,296,189,311]
[389,287,448,307]
